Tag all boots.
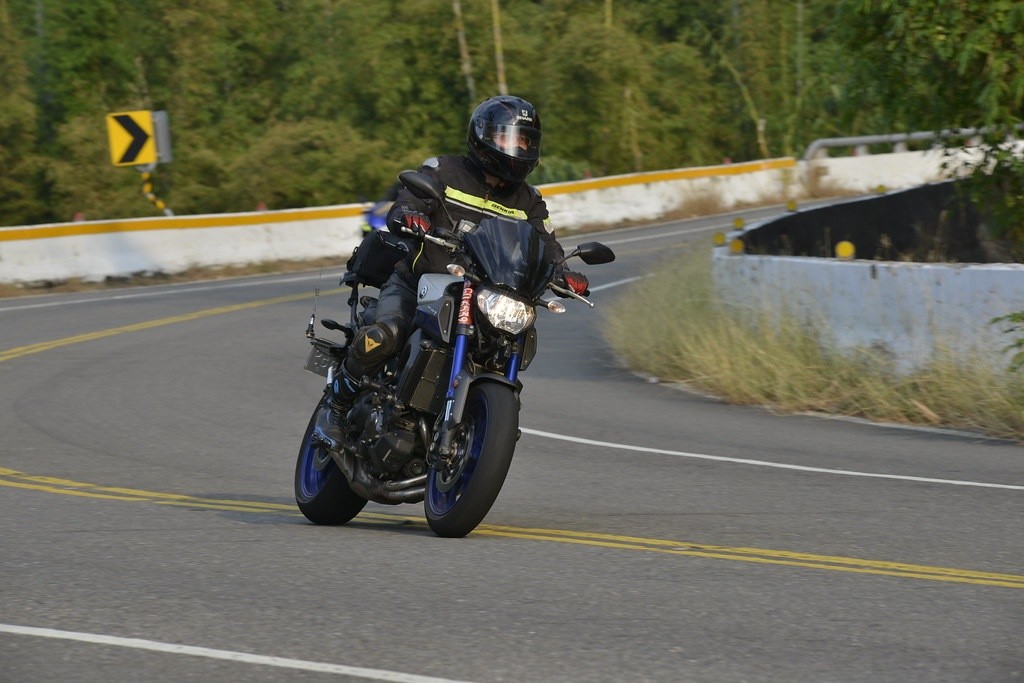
[315,359,364,456]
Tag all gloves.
[557,271,589,299]
[392,210,431,238]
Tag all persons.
[312,93,589,452]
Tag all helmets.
[467,95,542,183]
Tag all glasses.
[490,132,531,146]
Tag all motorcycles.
[291,171,617,538]
